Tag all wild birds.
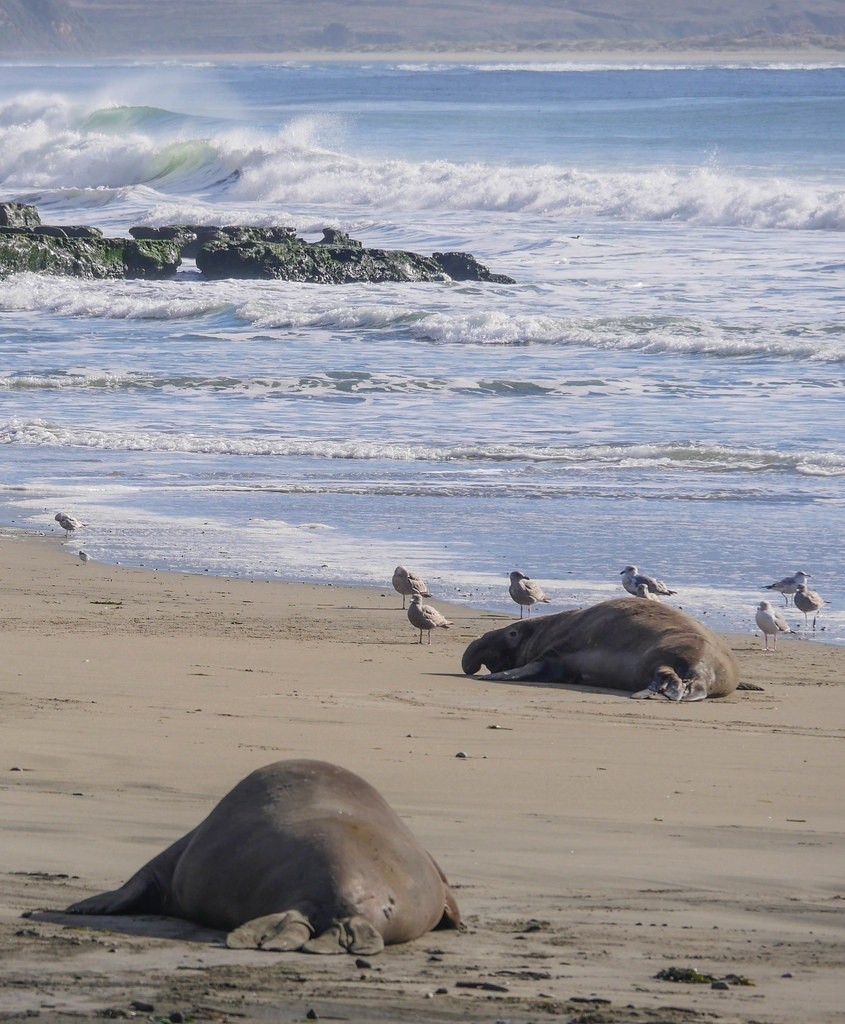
[79,551,92,565]
[390,563,832,656]
[53,512,90,540]
